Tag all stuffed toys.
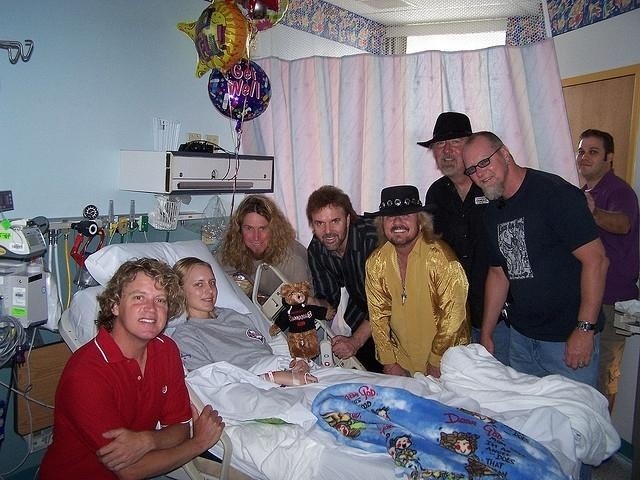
[269,280,336,360]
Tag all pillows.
[85,239,252,326]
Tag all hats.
[363,185,429,217]
[417,112,475,149]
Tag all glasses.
[464,147,501,176]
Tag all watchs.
[575,320,599,332]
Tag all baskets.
[148,196,181,231]
[153,118,181,153]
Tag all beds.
[57,238,623,480]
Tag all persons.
[575,129,640,415]
[364,185,472,378]
[213,195,316,306]
[417,112,510,367]
[463,131,610,391]
[37,257,225,479]
[307,186,386,374]
[169,258,318,387]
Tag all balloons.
[177,0,289,121]
